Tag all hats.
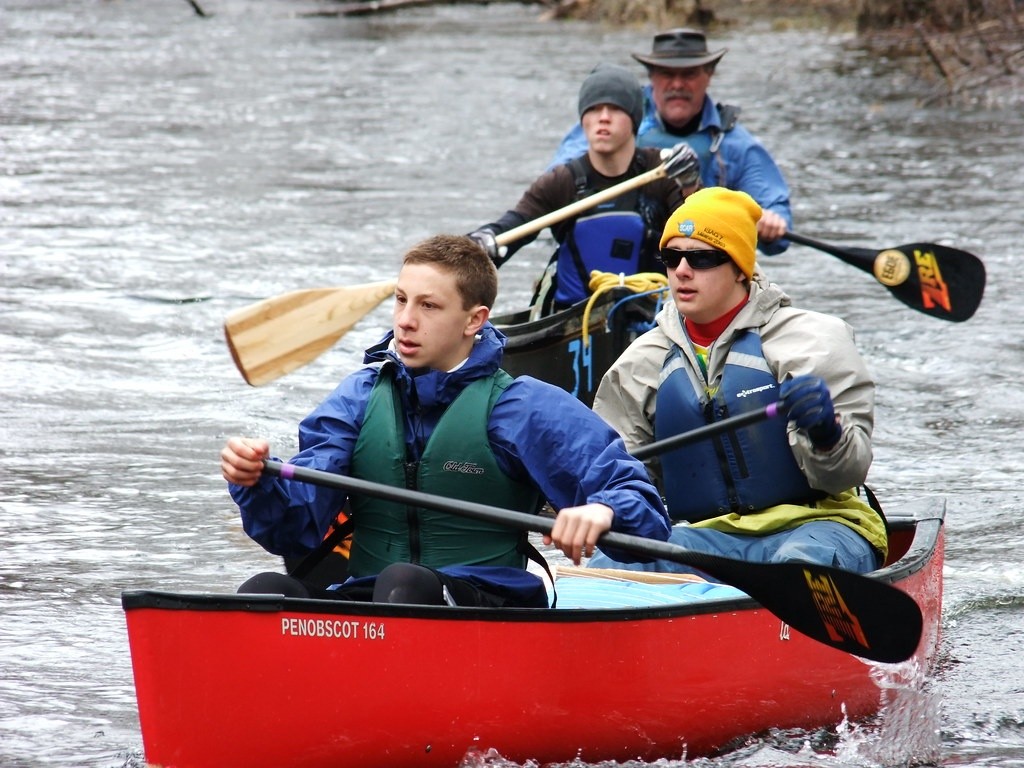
[632,30,728,69]
[578,62,643,135]
[659,186,762,280]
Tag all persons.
[468,62,705,407]
[548,28,794,256]
[587,186,890,577]
[221,236,674,607]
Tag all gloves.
[465,226,498,258]
[659,142,703,187]
[778,374,842,452]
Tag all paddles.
[626,398,789,460]
[783,231,987,324]
[261,458,923,664]
[222,160,667,387]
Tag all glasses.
[661,248,731,270]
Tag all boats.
[117,491,957,768]
[477,266,672,409]
